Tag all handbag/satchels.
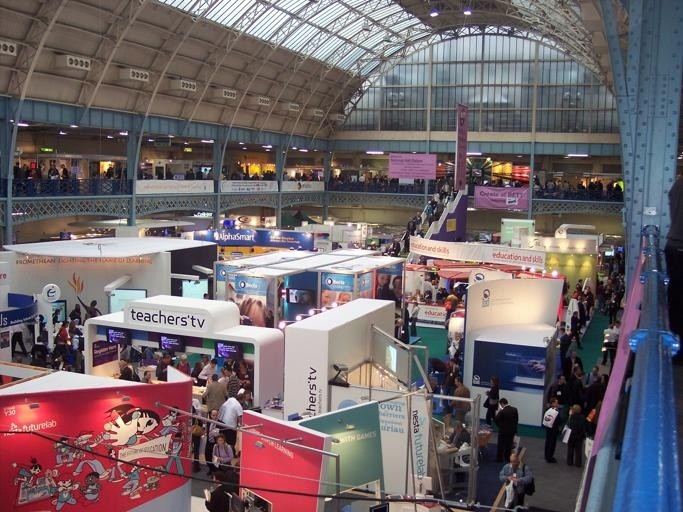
[524,476,536,497]
[560,425,572,444]
[191,419,202,437]
[504,480,515,508]
[489,398,497,405]
[543,408,559,428]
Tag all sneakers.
[544,456,557,463]
[567,461,582,467]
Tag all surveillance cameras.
[329,364,348,386]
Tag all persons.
[319,290,337,306]
[138,171,153,179]
[186,168,216,180]
[540,262,627,469]
[283,171,319,181]
[13,162,69,192]
[231,169,277,180]
[112,346,251,511]
[201,291,276,329]
[7,296,102,376]
[157,168,164,180]
[376,204,448,263]
[166,168,173,180]
[335,292,349,307]
[326,164,625,204]
[106,167,114,178]
[373,274,535,512]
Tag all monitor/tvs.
[51,300,67,323]
[106,327,132,345]
[286,288,314,305]
[215,340,243,360]
[159,332,185,352]
[200,166,211,174]
[181,278,208,299]
[108,289,147,313]
